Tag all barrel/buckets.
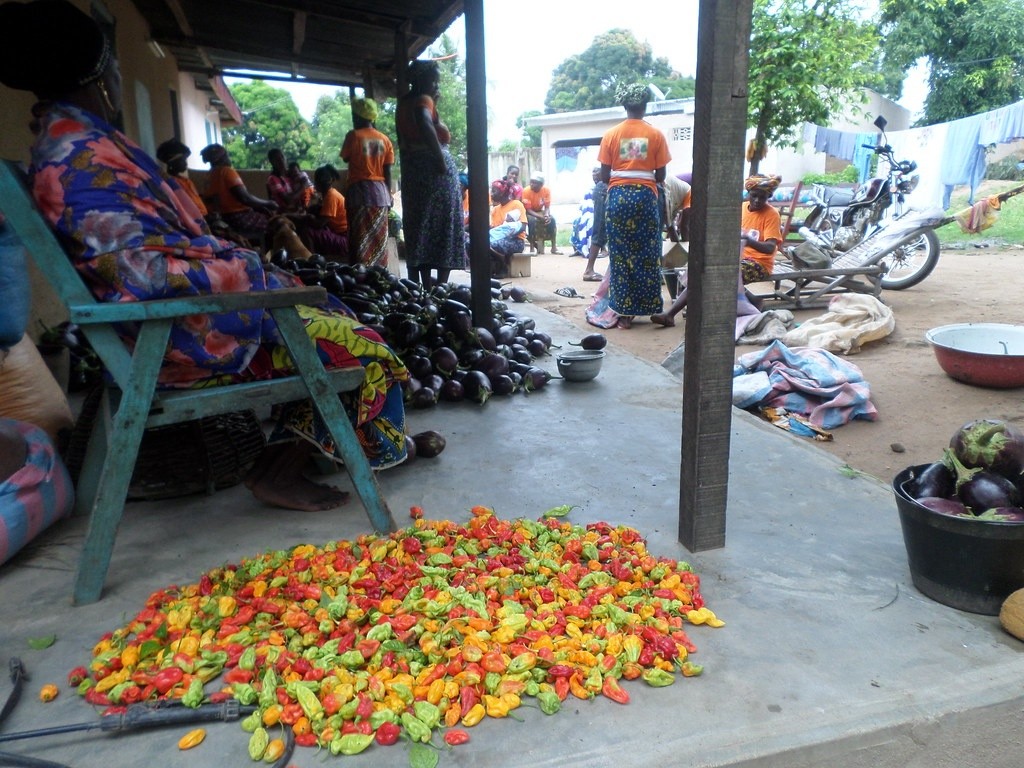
[662,268,681,298]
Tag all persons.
[396,60,466,288]
[340,97,396,267]
[154,139,251,246]
[659,173,691,302]
[285,164,348,255]
[597,81,672,328]
[569,168,608,258]
[0,0,411,512]
[490,165,528,280]
[202,144,279,231]
[583,182,608,282]
[265,149,317,215]
[649,173,783,327]
[522,177,563,255]
[457,173,470,266]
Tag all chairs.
[756,205,955,310]
[0,159,400,608]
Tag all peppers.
[40,504,727,762]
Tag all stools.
[509,253,532,278]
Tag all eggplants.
[907,418,1024,523]
[271,246,610,461]
[35,316,103,386]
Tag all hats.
[201,144,225,164]
[351,98,378,120]
[404,59,438,85]
[0,0,111,95]
[156,137,190,162]
[530,171,544,184]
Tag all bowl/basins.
[556,349,606,382]
[925,322,1024,390]
[892,464,1024,615]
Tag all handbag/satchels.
[788,240,830,271]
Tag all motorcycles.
[790,114,940,290]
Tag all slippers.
[597,251,609,258]
[615,323,626,328]
[583,273,603,281]
[555,286,584,298]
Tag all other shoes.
[551,250,564,255]
